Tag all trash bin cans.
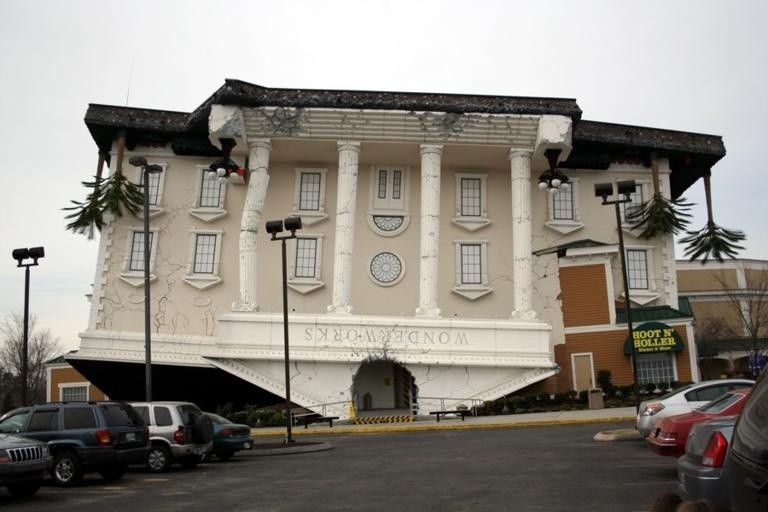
[364,393,371,410]
[588,388,603,410]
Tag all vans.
[0,400,254,502]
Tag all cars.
[636,361,767,512]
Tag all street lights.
[128,155,164,400]
[265,216,303,444]
[11,246,45,406]
[594,179,640,412]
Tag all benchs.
[283,407,318,425]
[429,409,470,422]
[304,416,339,429]
